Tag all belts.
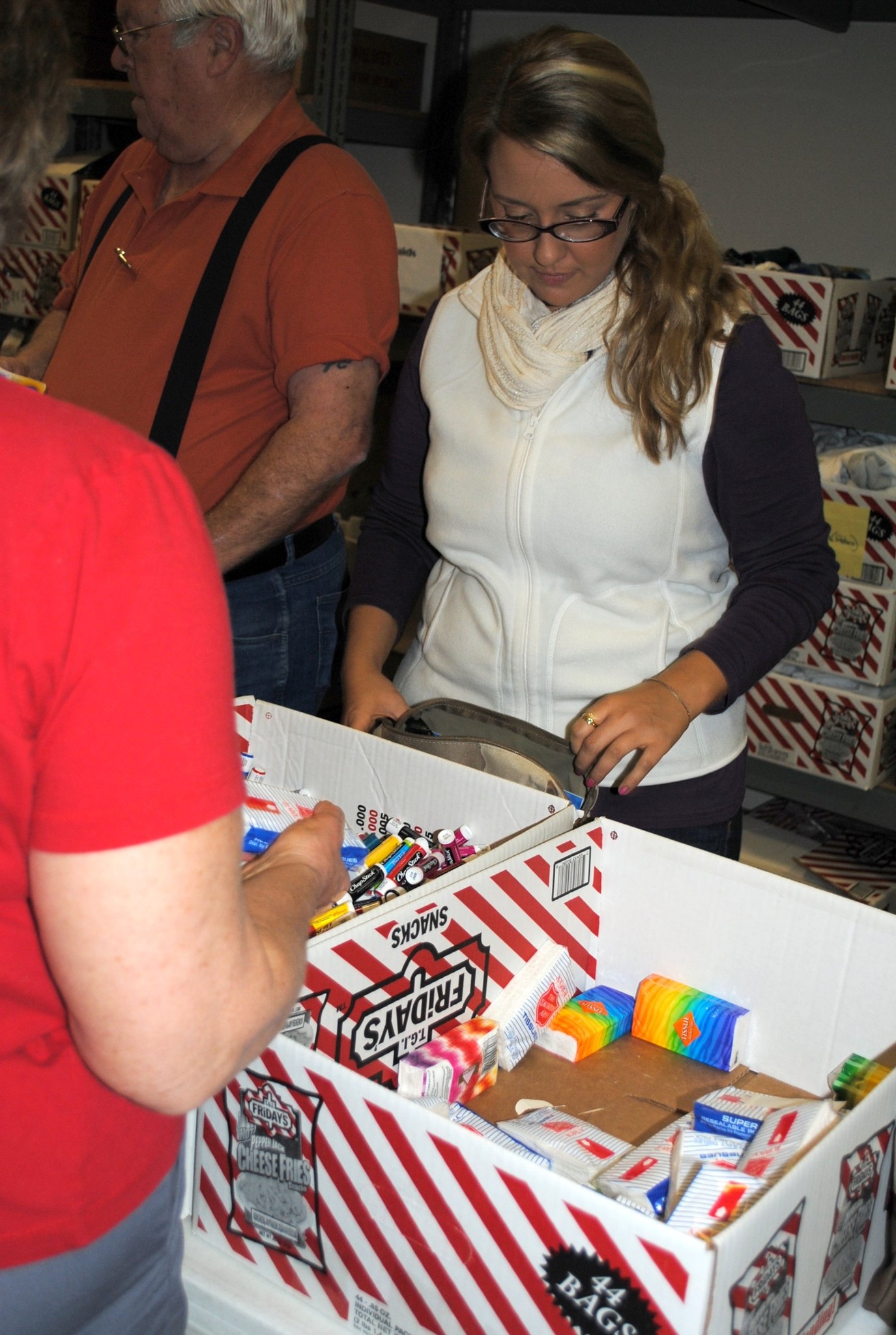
[222,514,340,584]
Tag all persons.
[0,0,399,734]
[346,38,839,860]
[0,376,351,1335]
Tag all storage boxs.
[1,150,108,321]
[290,15,427,111]
[179,222,896,1335]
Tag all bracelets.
[642,677,691,725]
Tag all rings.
[581,712,598,729]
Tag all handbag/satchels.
[379,698,601,822]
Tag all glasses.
[112,16,193,58]
[478,180,628,245]
